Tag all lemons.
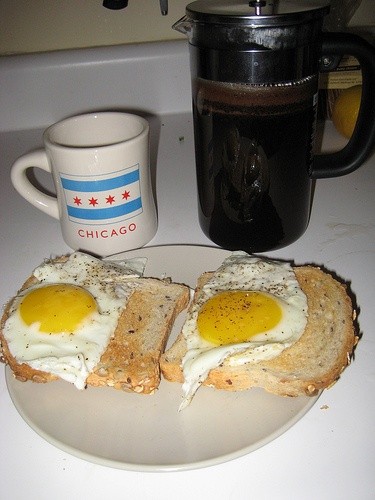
[333,83,362,138]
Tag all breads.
[0,254,189,392]
[157,266,360,397]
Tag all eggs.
[177,251,307,412]
[2,251,146,391]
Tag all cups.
[10,111,160,257]
[173,0,374,252]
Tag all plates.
[5,243,324,471]
